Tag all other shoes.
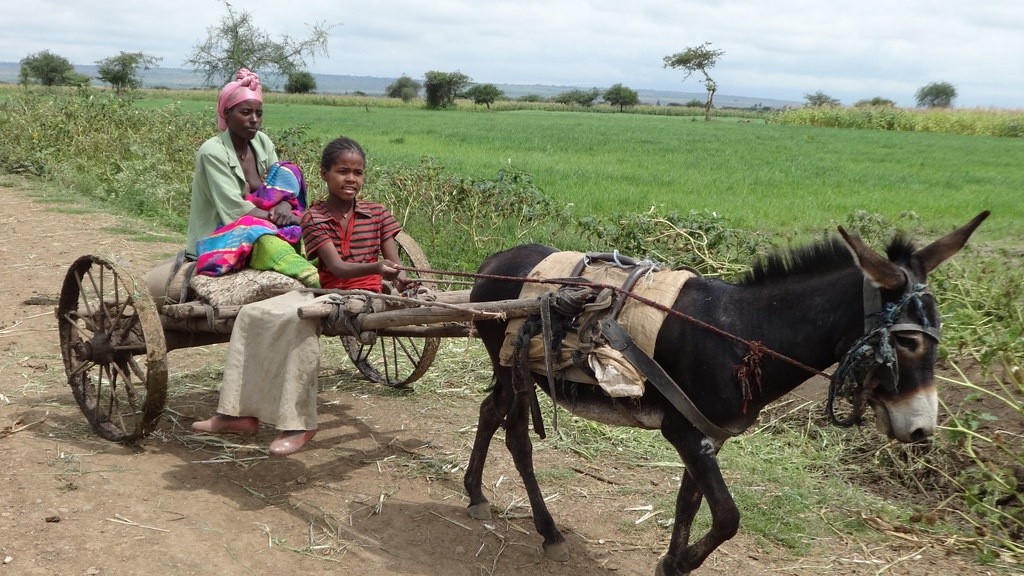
[268,421,316,457]
[191,413,260,435]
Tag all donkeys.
[464,207,993,576]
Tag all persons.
[186,68,405,457]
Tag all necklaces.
[327,200,352,218]
[234,143,247,160]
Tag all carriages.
[53,209,991,576]
